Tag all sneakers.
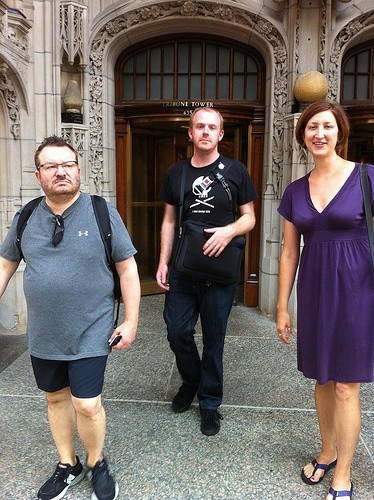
[199,396,224,435]
[173,382,200,412]
[36,455,85,500]
[86,450,119,500]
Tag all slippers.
[329,481,353,500]
[301,459,337,485]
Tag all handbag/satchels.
[171,220,246,286]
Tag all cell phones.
[108,334,121,346]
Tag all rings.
[287,328,291,330]
[278,334,281,336]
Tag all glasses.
[51,215,65,247]
[38,161,78,172]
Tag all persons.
[276,102,374,500]
[155,108,256,436]
[0,137,141,500]
